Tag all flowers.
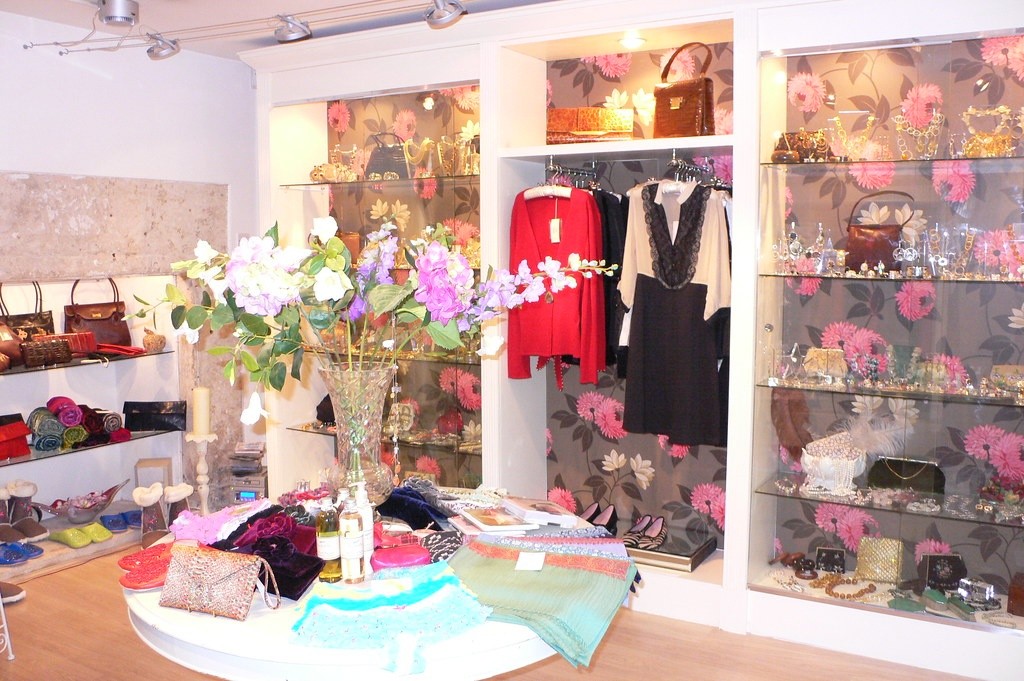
[119,217,618,424]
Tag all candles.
[190,386,210,433]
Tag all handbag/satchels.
[367,133,415,180]
[546,107,634,144]
[0,280,187,455]
[774,129,834,162]
[654,42,715,140]
[802,348,848,378]
[856,537,903,584]
[335,233,362,264]
[918,553,968,590]
[990,365,1024,392]
[844,191,915,272]
[157,507,326,622]
[867,456,946,495]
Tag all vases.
[318,361,398,503]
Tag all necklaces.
[962,105,1011,144]
[788,220,825,260]
[405,138,433,166]
[1005,224,1024,265]
[439,142,458,176]
[883,459,928,479]
[886,346,921,385]
[945,494,978,520]
[801,456,856,496]
[333,150,357,173]
[892,112,944,160]
[928,227,977,277]
[834,115,874,160]
[810,572,893,603]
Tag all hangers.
[525,164,622,206]
[630,159,727,206]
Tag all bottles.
[314,483,374,584]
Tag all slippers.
[100,510,143,532]
[51,522,112,548]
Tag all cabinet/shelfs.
[0,282,178,584]
[250,1,1024,681]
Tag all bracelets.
[774,477,798,493]
[872,486,921,505]
[854,487,874,506]
[906,502,941,512]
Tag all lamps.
[276,15,312,43]
[98,1,138,26]
[425,2,465,29]
[147,34,180,60]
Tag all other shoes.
[0,581,26,603]
[117,541,171,591]
[622,515,668,551]
[579,502,619,534]
[0,542,44,565]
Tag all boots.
[0,479,51,545]
[132,483,194,550]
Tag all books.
[448,495,574,536]
